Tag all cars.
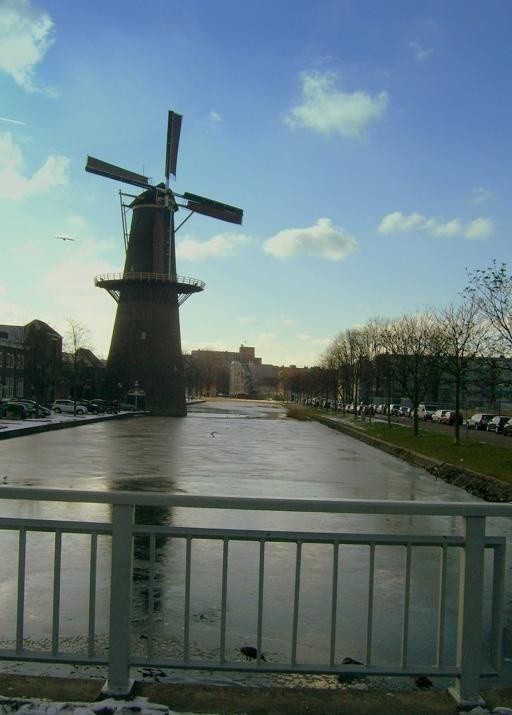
[376,402,511,437]
[305,397,376,414]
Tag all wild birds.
[340,657,366,666]
[55,236,74,242]
[412,675,434,691]
[238,646,267,662]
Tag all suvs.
[0,398,137,419]
